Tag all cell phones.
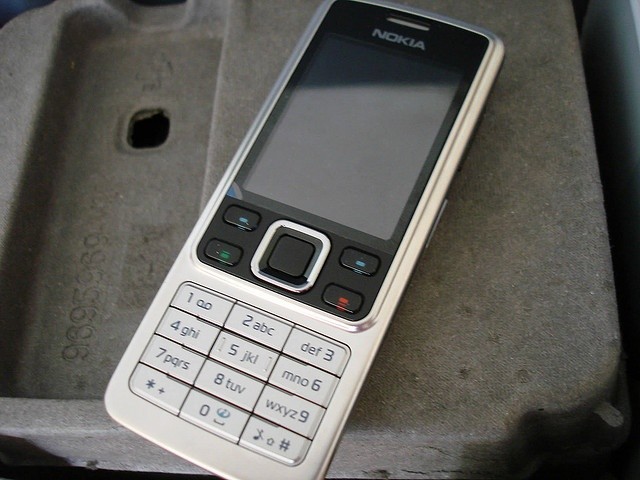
[102,0,507,480]
